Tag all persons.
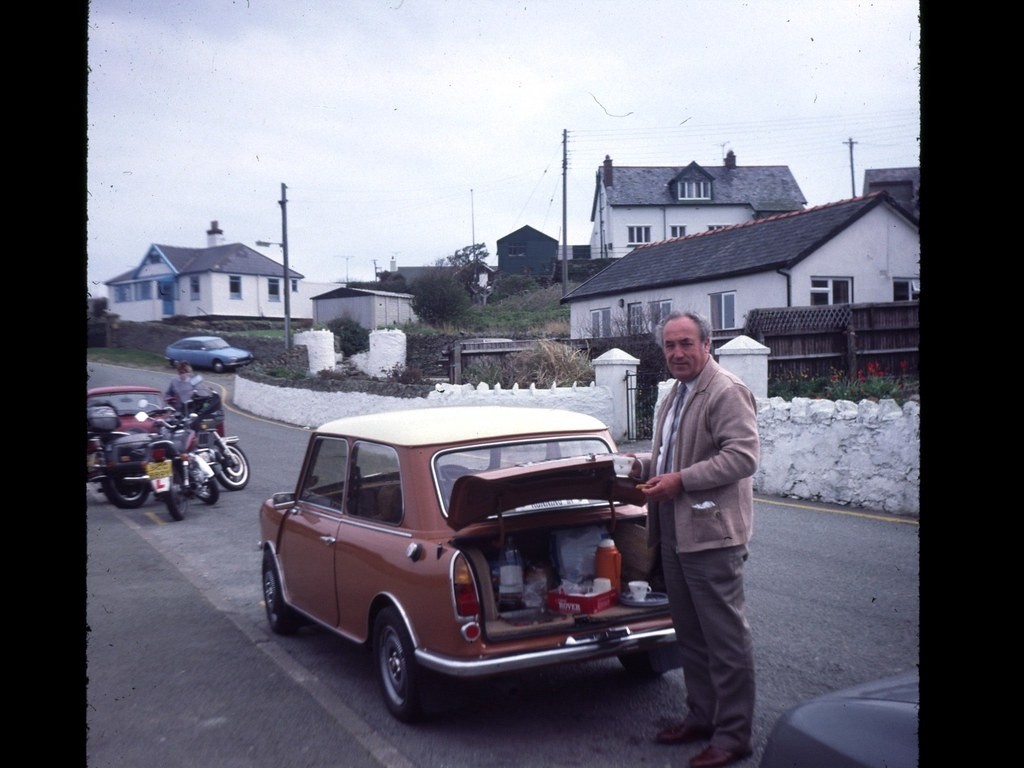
[621,310,758,767]
[166,361,209,414]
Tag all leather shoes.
[656,726,715,744]
[689,745,754,768]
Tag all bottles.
[498,536,523,603]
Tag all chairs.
[373,484,402,523]
[439,465,475,506]
[140,395,159,412]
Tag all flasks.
[596,534,622,598]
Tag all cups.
[628,580,650,603]
[593,578,611,595]
[613,455,635,478]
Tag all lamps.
[618,298,624,308]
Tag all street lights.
[256,240,290,349]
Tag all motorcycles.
[85,376,251,521]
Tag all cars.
[256,406,683,725]
[165,335,254,373]
[759,671,919,768]
[87,387,225,458]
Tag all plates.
[622,591,669,606]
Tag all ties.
[658,383,687,475]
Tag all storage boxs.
[546,585,617,615]
